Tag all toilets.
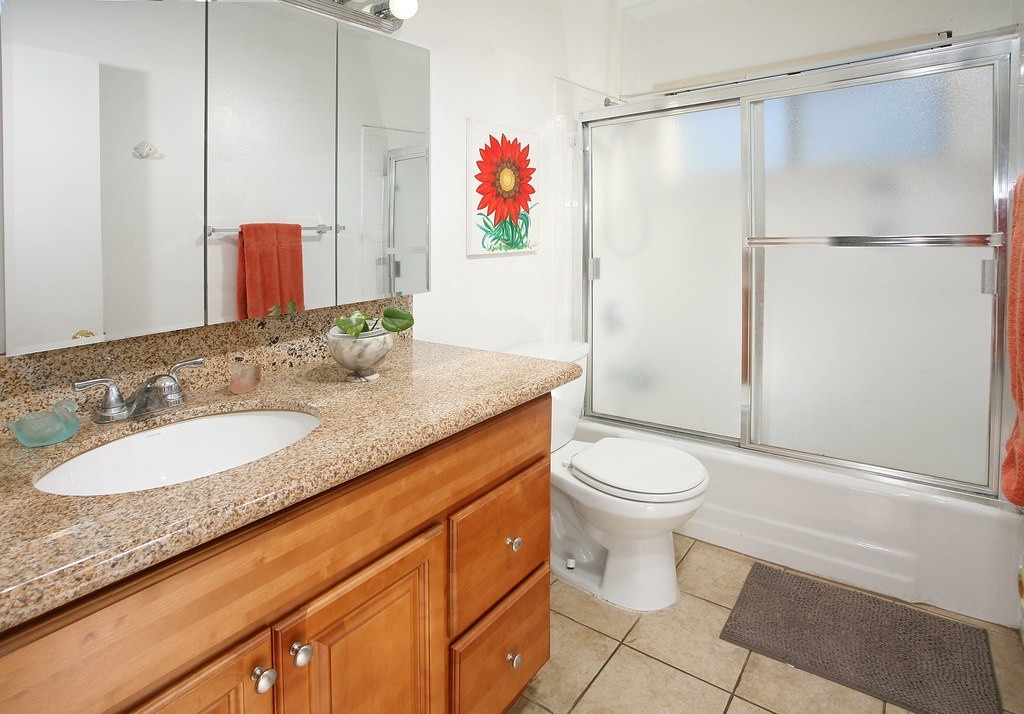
[527,339,712,613]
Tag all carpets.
[718,562,1003,714]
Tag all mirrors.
[0,0,431,362]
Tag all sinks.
[31,395,323,498]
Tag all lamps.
[369,0,418,31]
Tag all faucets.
[69,356,208,425]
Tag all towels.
[1000,172,1024,504]
[236,222,305,318]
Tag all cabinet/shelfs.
[0,393,559,714]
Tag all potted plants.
[328,307,414,387]
